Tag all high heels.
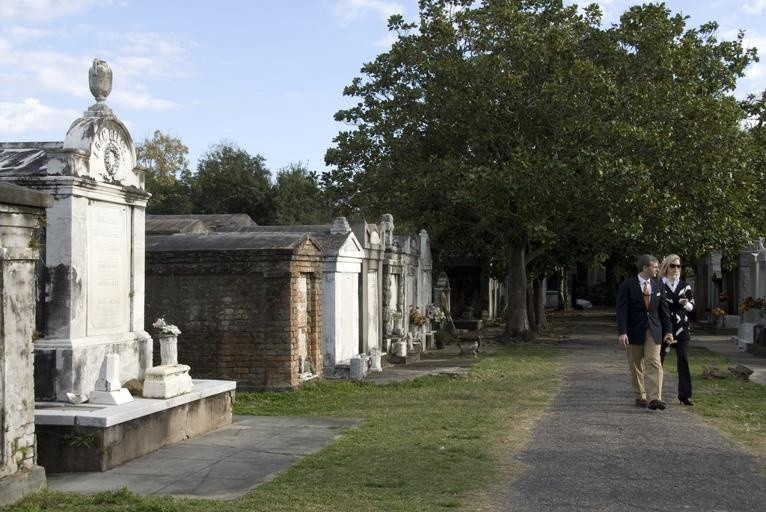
[678,396,694,406]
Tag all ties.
[642,280,650,311]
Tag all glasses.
[669,264,681,269]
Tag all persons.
[616,255,675,410]
[660,253,697,406]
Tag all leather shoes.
[648,400,665,410]
[636,399,648,407]
